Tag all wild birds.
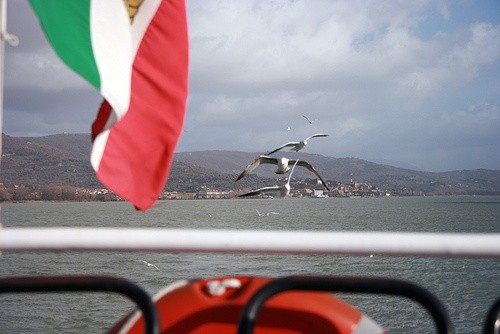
[208,114,330,217]
[133,260,160,271]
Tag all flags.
[27,0,190,213]
